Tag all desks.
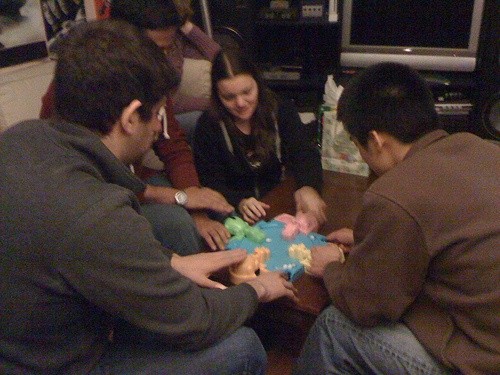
[193,170,376,339]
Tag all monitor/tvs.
[339,0,484,72]
